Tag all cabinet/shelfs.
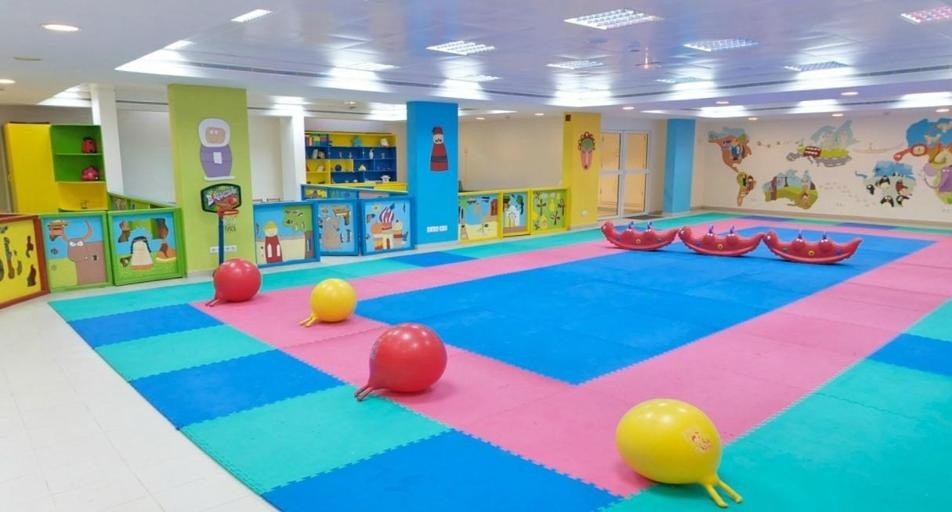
[49,123,108,211]
[307,130,397,187]
